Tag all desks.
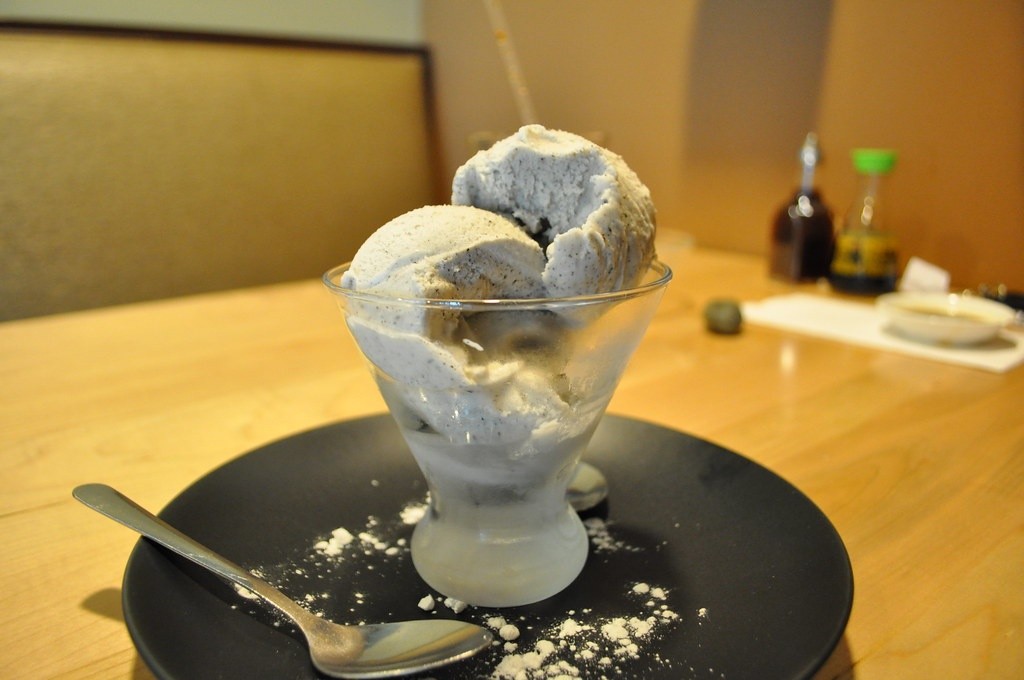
[0,242,1024,680]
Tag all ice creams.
[337,123,658,508]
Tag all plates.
[874,289,1012,343]
[122,405,854,680]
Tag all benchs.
[0,16,447,322]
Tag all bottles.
[823,148,899,294]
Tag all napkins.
[738,291,1023,374]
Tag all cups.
[324,257,672,607]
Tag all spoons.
[70,480,493,679]
